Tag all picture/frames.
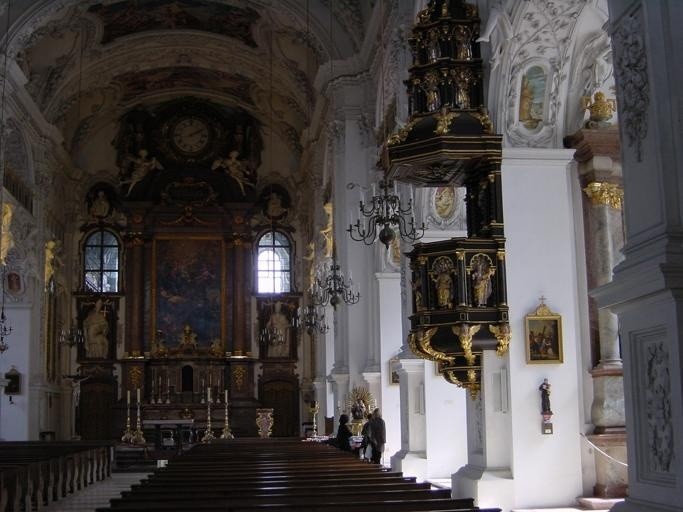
[525,297,563,364]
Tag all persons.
[336,413,353,451]
[473,1,513,43]
[519,76,533,124]
[265,301,290,356]
[319,202,335,258]
[350,399,366,418]
[538,378,552,412]
[434,187,455,220]
[115,148,159,201]
[430,264,454,309]
[471,264,491,309]
[370,407,387,463]
[264,191,287,217]
[44,240,57,288]
[528,326,557,360]
[221,150,255,196]
[424,72,439,112]
[452,69,469,107]
[360,412,373,455]
[88,191,113,218]
[0,202,17,267]
[81,299,110,362]
[301,239,315,290]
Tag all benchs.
[1,443,118,510]
[109,438,502,511]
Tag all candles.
[127,372,231,404]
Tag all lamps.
[345,161,429,257]
[60,317,84,351]
[260,322,286,347]
[290,300,330,337]
[312,238,363,311]
[0,264,12,356]
